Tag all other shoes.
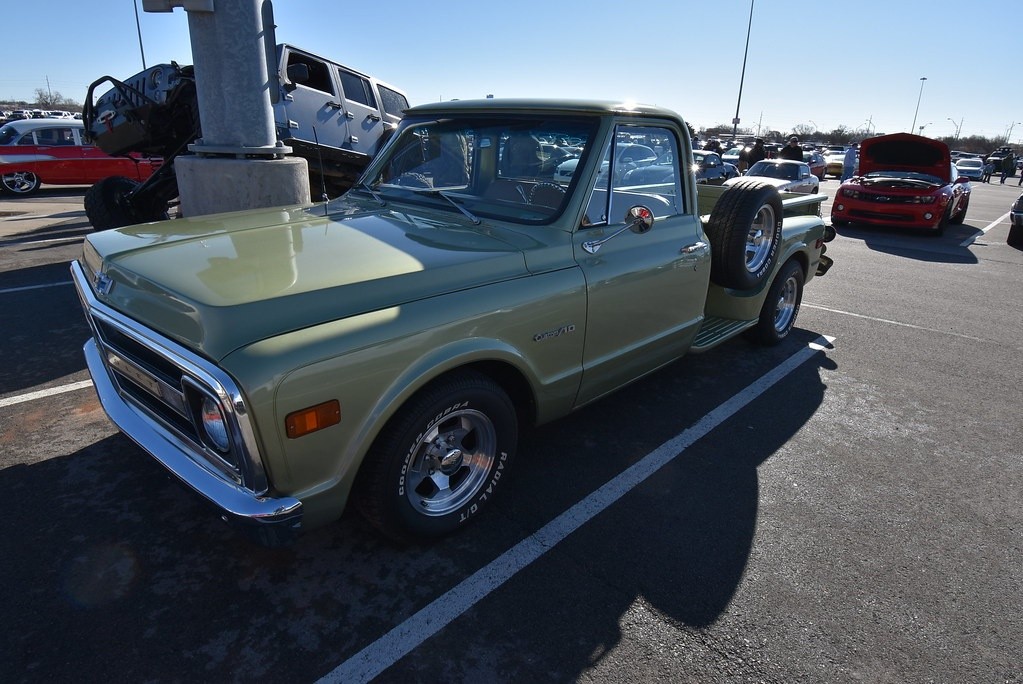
[981,181,1023,186]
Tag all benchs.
[484,177,677,223]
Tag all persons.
[644,134,655,151]
[501,129,543,179]
[692,137,702,163]
[1000,153,1013,184]
[780,136,803,162]
[1018,169,1023,186]
[983,160,995,183]
[704,140,766,172]
[622,133,632,143]
[840,142,859,184]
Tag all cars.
[0,108,86,133]
[0,118,170,197]
[418,121,671,195]
[622,150,743,185]
[947,145,1023,183]
[720,158,819,200]
[67,99,836,539]
[831,133,971,239]
[691,128,865,179]
[1010,194,1023,226]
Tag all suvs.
[79,42,414,230]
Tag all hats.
[789,136,800,142]
[756,138,765,144]
[1008,153,1013,156]
[989,159,993,162]
